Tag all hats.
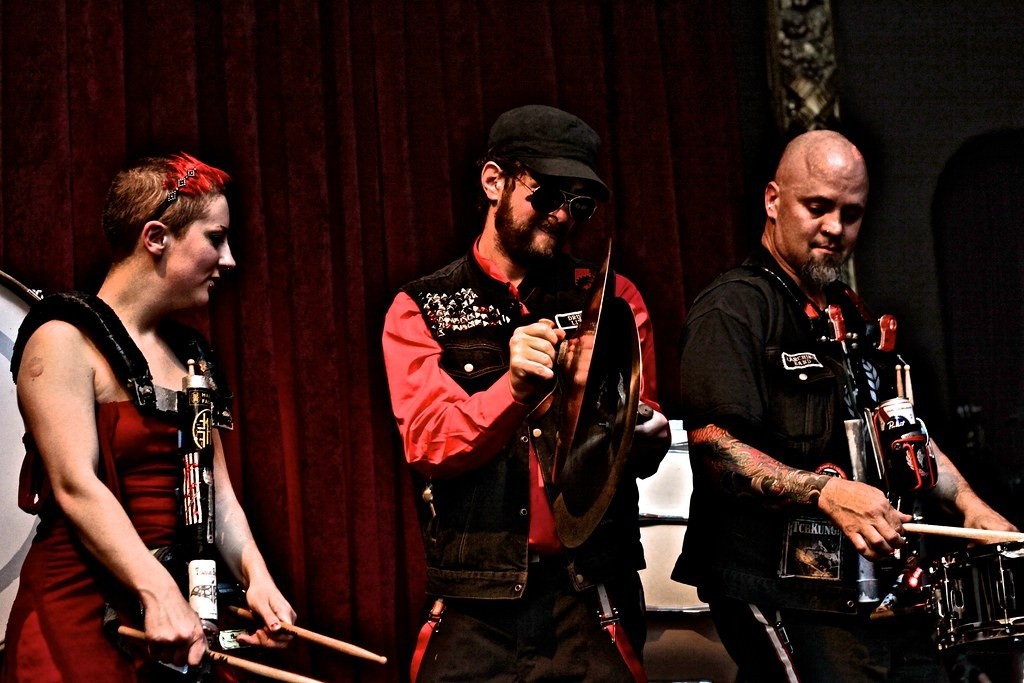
[491,105,608,194]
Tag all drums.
[929,540,1024,656]
[0,269,59,656]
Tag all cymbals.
[528,232,644,548]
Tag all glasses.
[514,175,598,224]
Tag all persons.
[0,152,297,683]
[381,105,670,683]
[671,131,1020,683]
